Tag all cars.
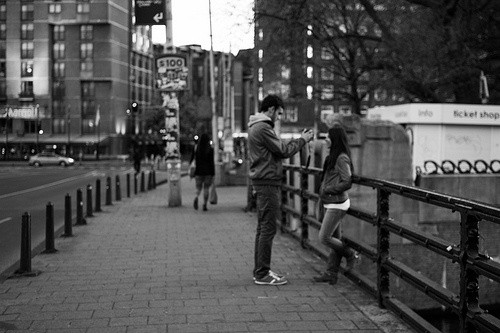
[0,144,35,161]
[28,150,74,168]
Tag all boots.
[311,255,341,284]
[340,244,362,273]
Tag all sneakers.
[255,274,287,285]
[252,270,283,280]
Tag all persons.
[312,124,358,285]
[246,94,314,286]
[189,133,215,211]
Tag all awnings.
[0,132,108,144]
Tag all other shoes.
[202,204,208,211]
[194,197,199,210]
